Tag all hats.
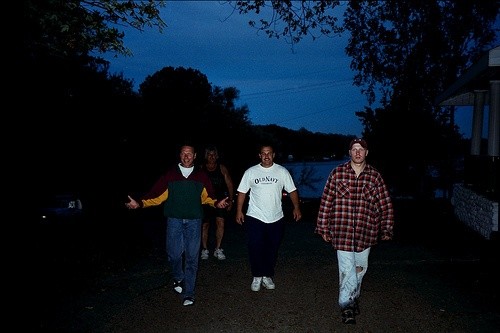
[350,138,367,148]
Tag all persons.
[315,136,395,324]
[199,147,233,261]
[235,144,301,291]
[125,142,229,306]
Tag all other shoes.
[342,297,361,324]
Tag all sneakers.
[251,276,263,292]
[262,276,275,289]
[201,249,209,260]
[214,249,226,260]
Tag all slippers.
[183,295,196,306]
[173,280,182,294]
